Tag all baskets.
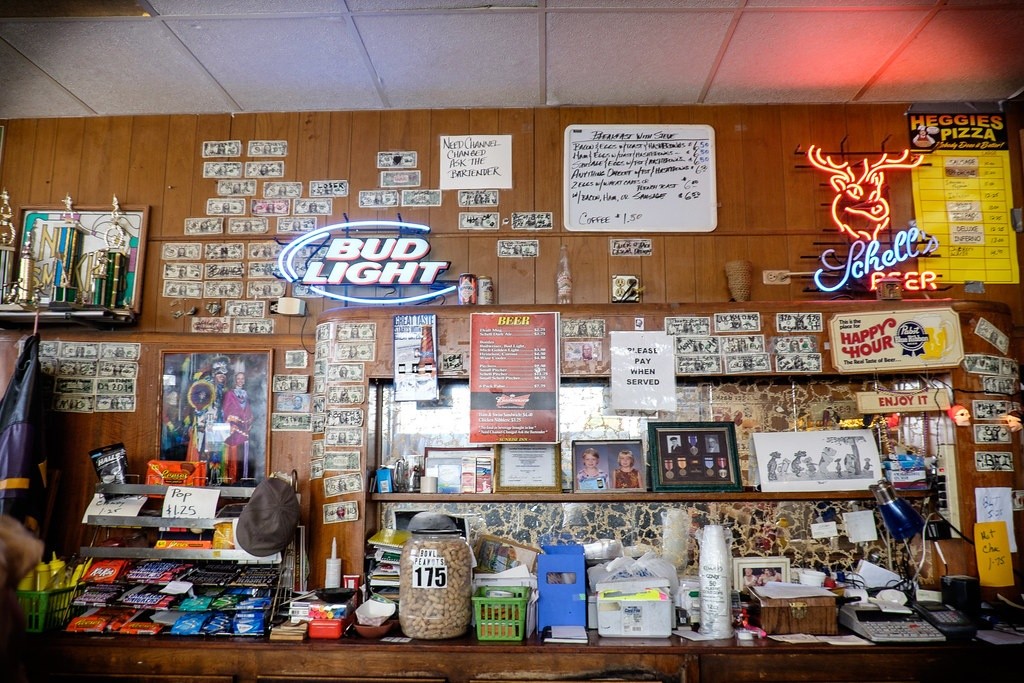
[472,587,531,641]
[16,582,92,633]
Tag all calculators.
[838,604,947,645]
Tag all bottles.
[556,244,573,305]
[15,551,91,621]
[417,324,439,409]
[324,536,342,588]
[688,591,700,626]
[399,528,472,641]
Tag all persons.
[742,568,757,586]
[707,438,720,452]
[611,450,642,489]
[669,437,682,452]
[576,448,610,492]
[758,568,781,584]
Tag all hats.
[236,479,302,557]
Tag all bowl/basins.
[316,588,357,604]
[354,621,394,638]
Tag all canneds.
[458,272,494,305]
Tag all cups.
[420,476,438,493]
[663,508,688,575]
[699,524,736,640]
[393,454,423,492]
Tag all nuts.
[400,539,473,639]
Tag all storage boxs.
[469,542,701,641]
[748,587,839,635]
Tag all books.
[270,621,310,641]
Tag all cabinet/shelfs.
[52,471,301,637]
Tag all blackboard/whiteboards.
[564,123,718,233]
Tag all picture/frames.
[156,345,276,484]
[571,439,647,494]
[732,557,790,593]
[492,443,562,495]
[647,421,744,493]
[734,558,791,595]
[9,204,149,315]
